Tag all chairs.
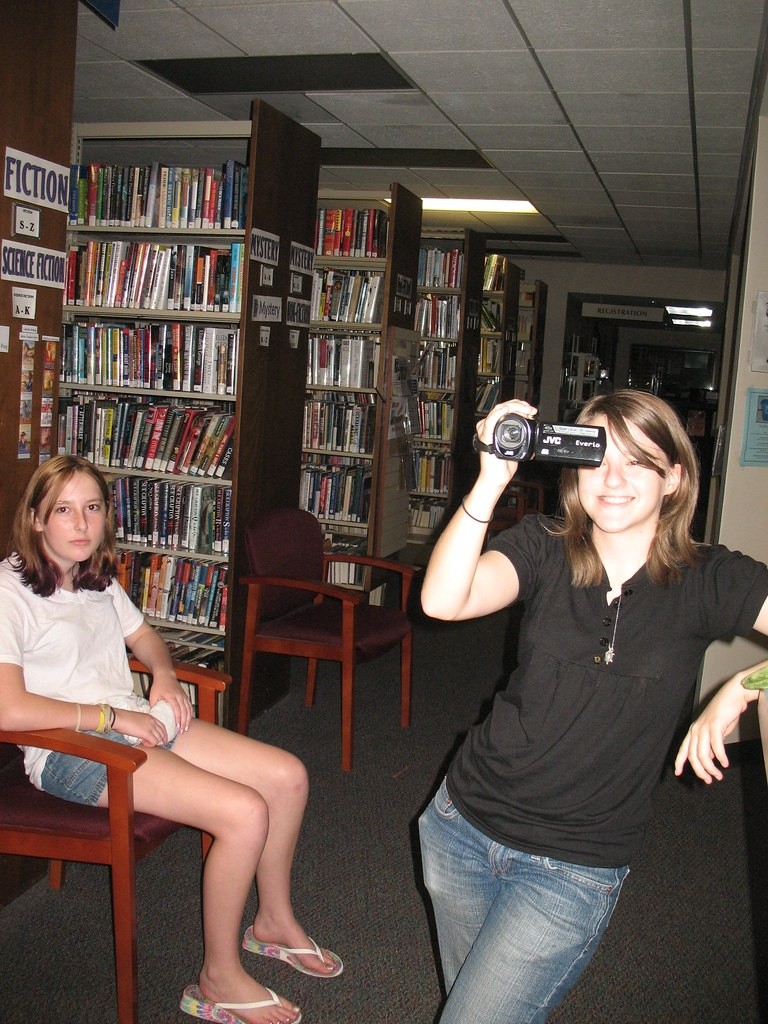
[485,481,546,545]
[237,506,423,772]
[0,657,232,1024]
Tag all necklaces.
[605,585,621,665]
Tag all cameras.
[493,413,607,468]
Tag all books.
[476,254,597,414]
[409,248,463,528]
[58,160,248,668]
[301,208,388,584]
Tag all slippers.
[243,924,343,977]
[180,985,301,1024]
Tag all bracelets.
[75,703,116,732]
[462,495,493,523]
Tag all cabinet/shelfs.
[0,0,550,905]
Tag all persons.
[1,455,344,1024]
[417,390,768,1024]
